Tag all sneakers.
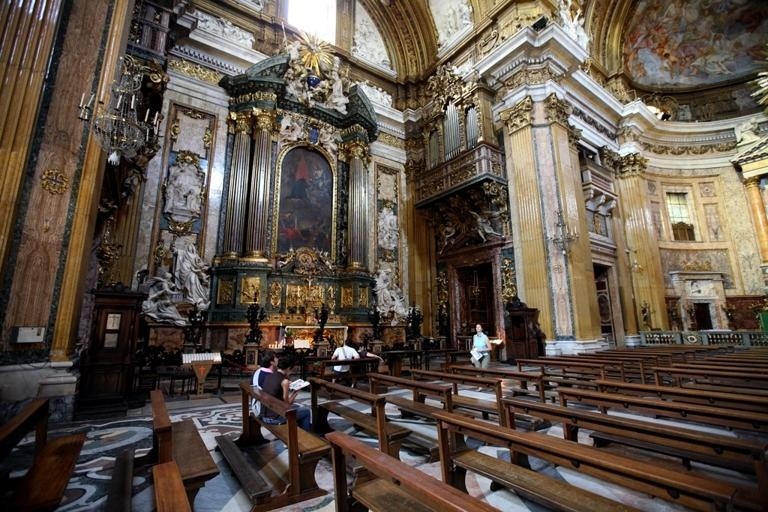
[283,444,287,449]
[272,437,279,440]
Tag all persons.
[329,338,361,388]
[317,214,333,251]
[165,170,185,214]
[355,340,386,377]
[469,322,495,394]
[311,164,326,191]
[279,211,297,252]
[251,349,279,417]
[280,41,349,162]
[143,239,210,327]
[307,216,322,249]
[468,208,503,244]
[181,183,202,215]
[260,354,311,433]
[385,219,401,241]
[630,1,768,76]
[374,264,409,327]
[375,211,388,238]
[439,221,460,255]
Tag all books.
[286,377,310,393]
[470,348,483,361]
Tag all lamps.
[624,249,644,273]
[546,210,580,256]
[74,54,160,168]
